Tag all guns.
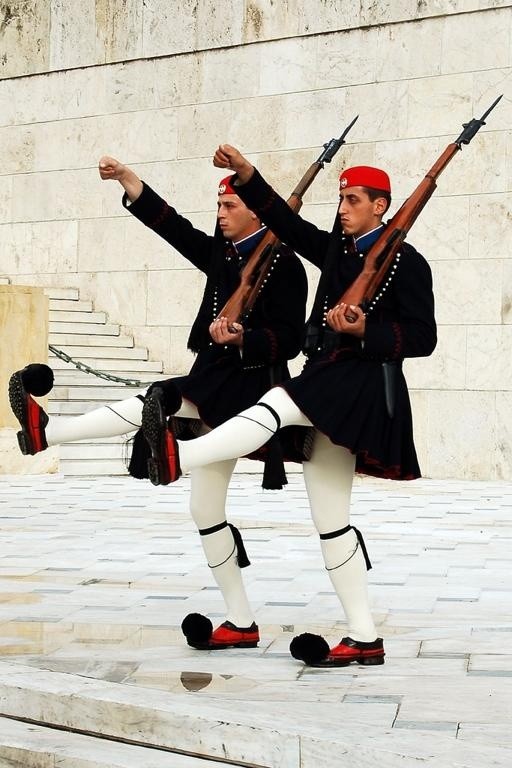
[333,94,503,324]
[216,115,358,333]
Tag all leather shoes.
[291,632,385,666]
[182,613,260,649]
[9,363,53,455]
[142,381,182,486]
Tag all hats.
[340,166,391,193]
[217,175,238,195]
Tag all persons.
[144,167,437,652]
[8,155,308,635]
